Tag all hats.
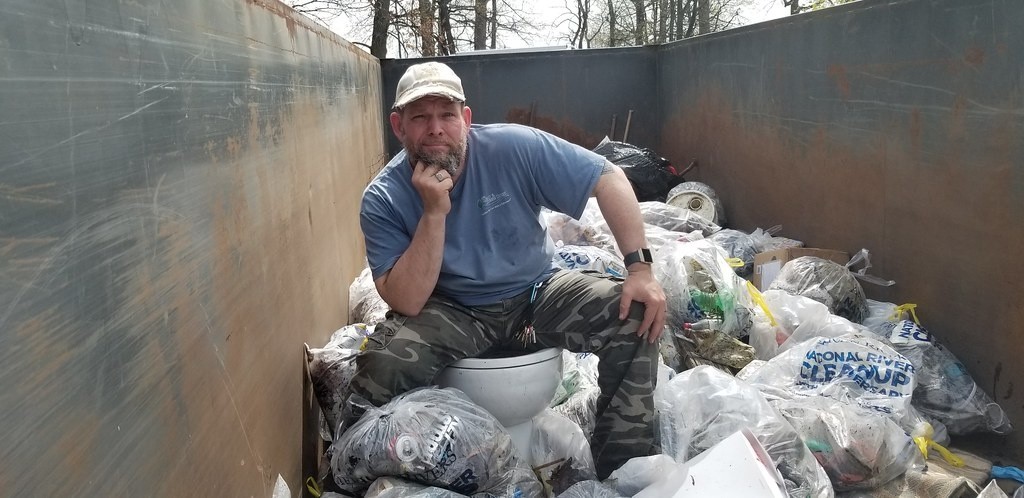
[391,61,467,110]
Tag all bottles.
[684,319,724,331]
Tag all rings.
[435,173,443,181]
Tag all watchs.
[623,248,654,270]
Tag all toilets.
[436,346,564,462]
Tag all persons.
[338,60,668,482]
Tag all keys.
[513,320,537,349]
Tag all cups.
[986,403,1011,435]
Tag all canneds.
[387,413,463,471]
